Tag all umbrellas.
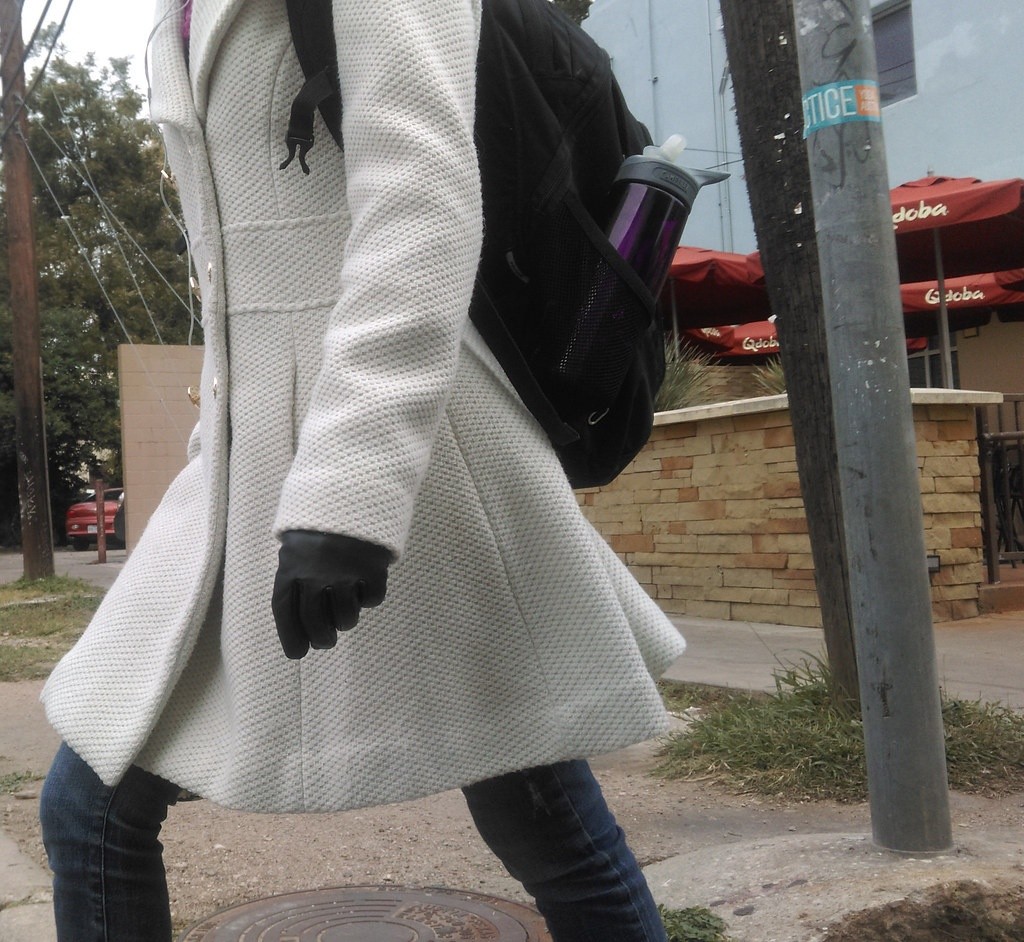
[887,174,1023,286]
[900,270,1023,336]
[652,246,771,366]
[709,322,928,367]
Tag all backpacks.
[277,0,667,490]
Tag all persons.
[38,0,687,942]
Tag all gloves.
[272,529,393,660]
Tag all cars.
[65,487,124,551]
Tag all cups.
[595,132,732,308]
